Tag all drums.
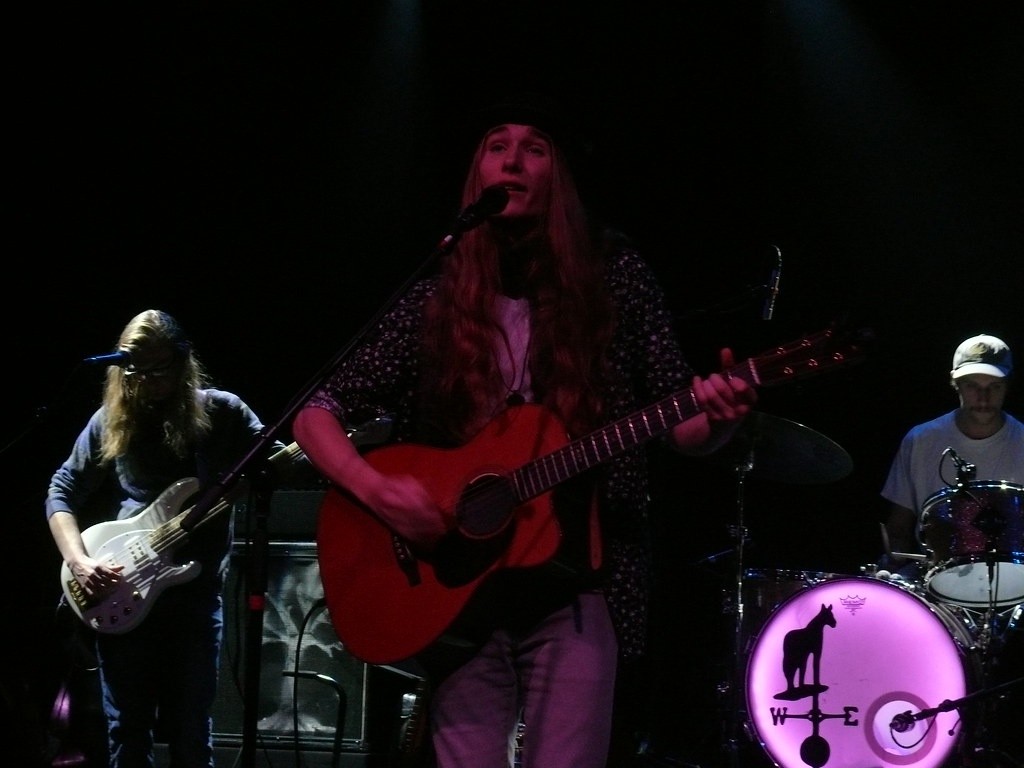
[741,577,983,768]
[722,566,855,653]
[919,478,1024,607]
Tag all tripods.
[685,462,757,768]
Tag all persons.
[293,123,756,768]
[46,310,309,768]
[880,334,1024,555]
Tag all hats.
[950,334,1013,384]
[476,104,563,151]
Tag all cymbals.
[715,412,851,486]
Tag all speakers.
[210,547,424,768]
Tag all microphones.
[83,350,134,368]
[765,256,782,320]
[948,447,977,480]
[451,185,510,236]
[890,713,915,733]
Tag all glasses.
[128,365,173,381]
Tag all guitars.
[57,410,391,635]
[315,321,878,689]
